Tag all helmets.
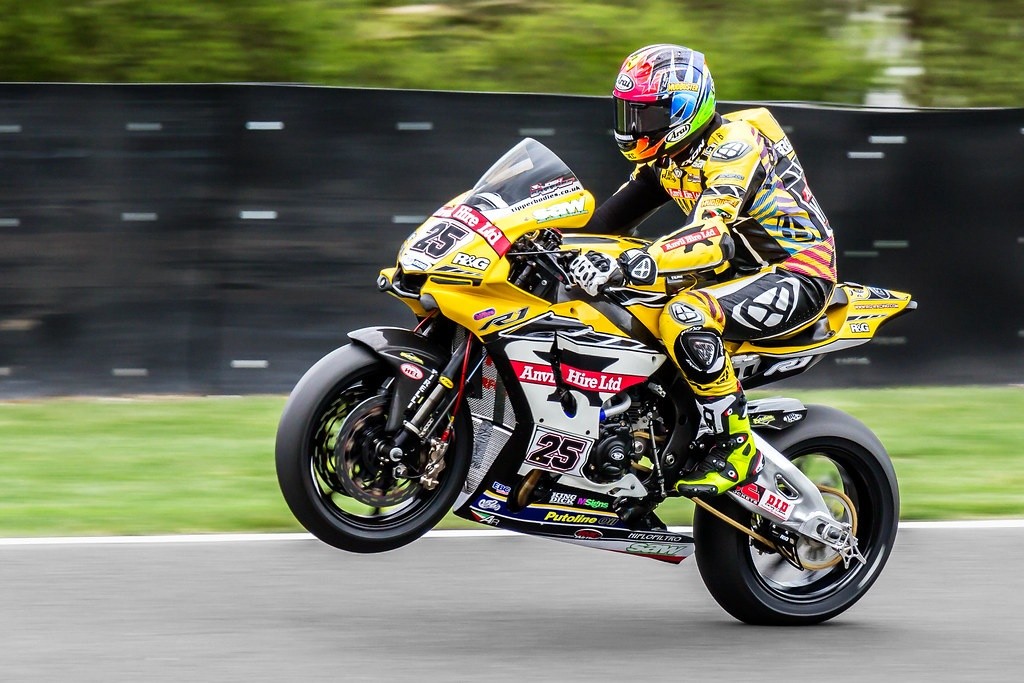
[613,44,716,164]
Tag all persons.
[559,44,837,497]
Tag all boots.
[677,378,765,498]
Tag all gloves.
[568,250,625,298]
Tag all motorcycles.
[274,134,920,625]
